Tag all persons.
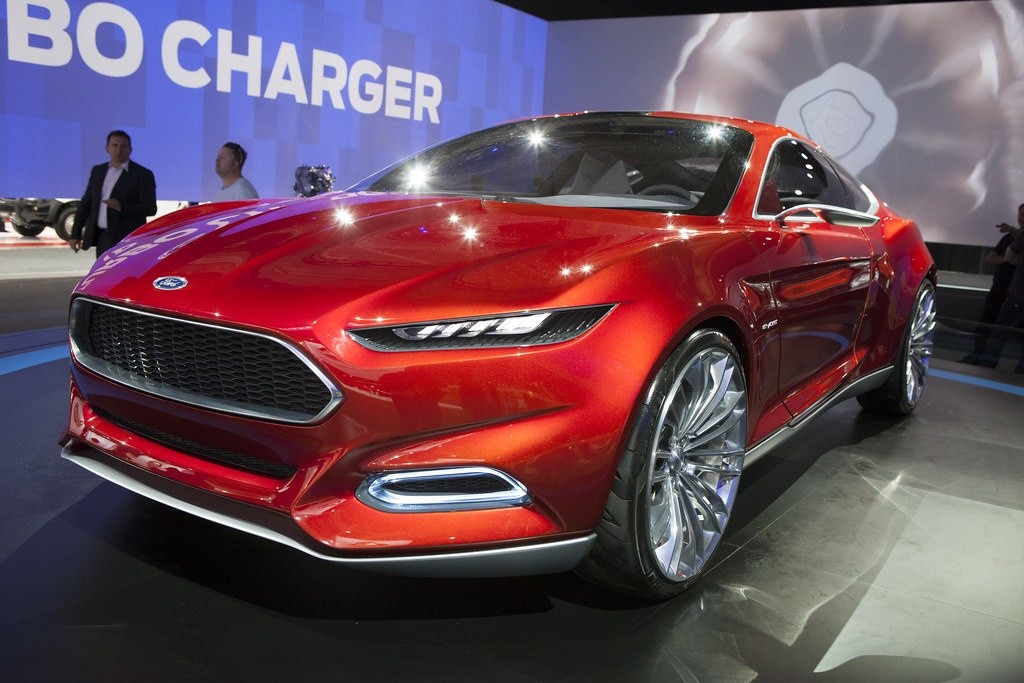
[212,142,259,202]
[69,129,157,259]
[969,203,1024,373]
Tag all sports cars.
[58,108,939,605]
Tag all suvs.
[0,196,89,243]
